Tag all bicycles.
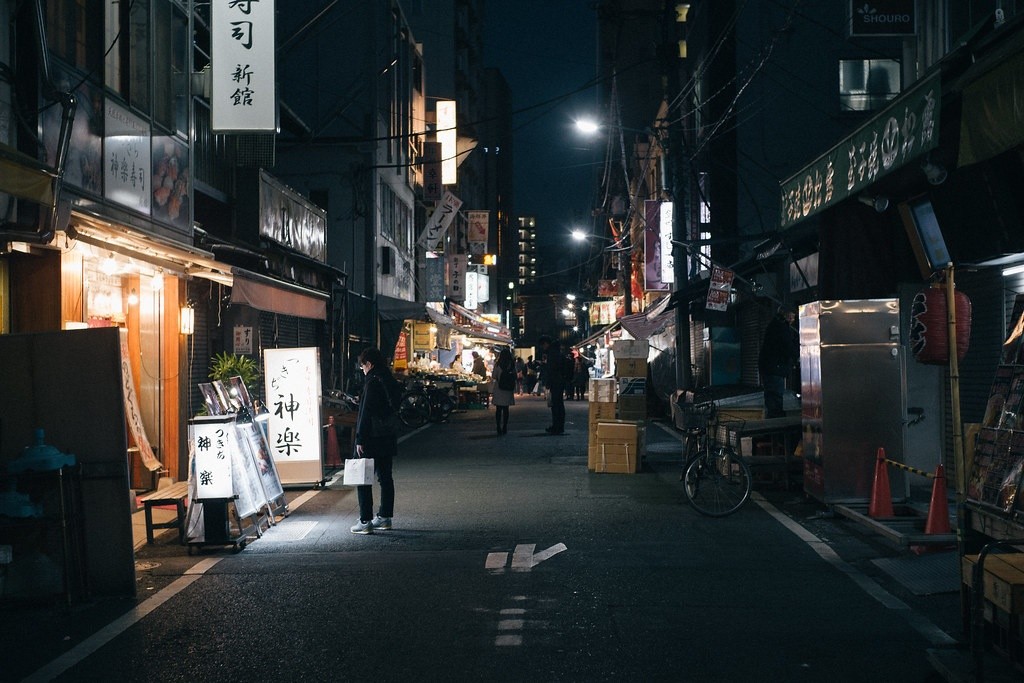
[679,385,752,518]
[395,374,457,429]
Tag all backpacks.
[497,361,516,391]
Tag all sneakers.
[371,513,392,530]
[351,517,372,535]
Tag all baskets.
[713,413,746,449]
[674,401,709,430]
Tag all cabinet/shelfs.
[712,416,804,492]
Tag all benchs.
[141,481,188,545]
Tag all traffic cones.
[869,448,895,518]
[907,463,958,552]
[322,415,345,470]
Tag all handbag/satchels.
[343,450,375,486]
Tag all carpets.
[136,496,187,510]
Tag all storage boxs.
[588,340,649,473]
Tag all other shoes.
[498,427,507,435]
[545,426,565,434]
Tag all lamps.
[223,400,253,426]
[181,307,194,334]
[254,399,271,421]
[857,189,906,214]
[921,152,948,185]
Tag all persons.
[535,355,547,396]
[762,306,798,418]
[492,349,516,437]
[351,347,400,534]
[539,335,563,434]
[451,355,463,372]
[472,352,486,380]
[515,355,536,396]
[565,354,593,402]
[415,353,438,368]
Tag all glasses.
[359,364,365,369]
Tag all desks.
[322,411,357,449]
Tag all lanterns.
[909,284,972,366]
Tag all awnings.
[621,306,673,362]
[69,208,330,320]
[952,28,1024,167]
[777,12,995,234]
[377,294,451,324]
[447,323,514,345]
[573,321,620,360]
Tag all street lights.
[570,112,693,393]
[570,228,632,317]
